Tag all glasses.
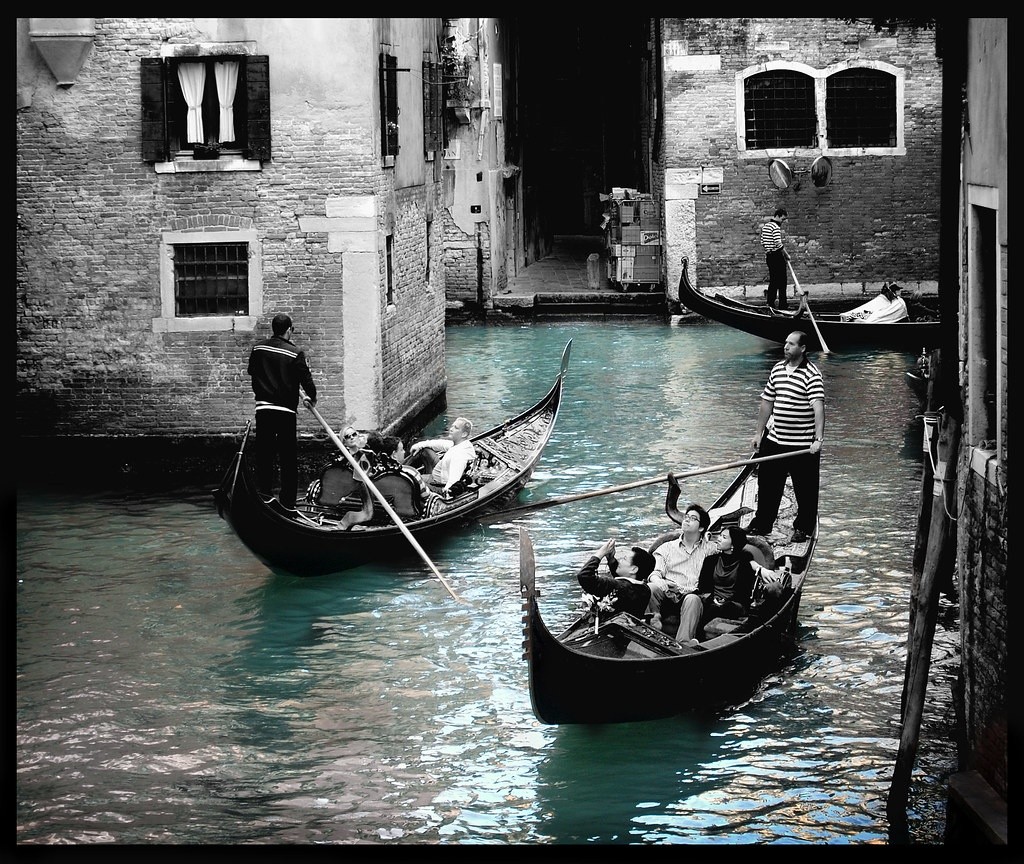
[683,514,700,524]
[344,432,358,439]
[289,327,294,332]
[621,556,631,563]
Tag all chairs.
[429,452,484,497]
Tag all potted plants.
[192,143,225,160]
[387,122,400,155]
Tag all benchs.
[307,448,420,518]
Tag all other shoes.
[740,525,768,537]
[791,527,811,544]
[683,638,699,646]
[781,306,795,310]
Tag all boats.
[214,339,576,579]
[520,445,822,727]
[677,255,942,352]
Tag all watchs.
[814,437,824,442]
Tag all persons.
[578,504,777,646]
[339,417,476,501]
[742,331,825,542]
[760,209,795,311]
[248,314,317,518]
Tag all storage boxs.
[608,188,661,282]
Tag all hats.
[889,284,903,291]
